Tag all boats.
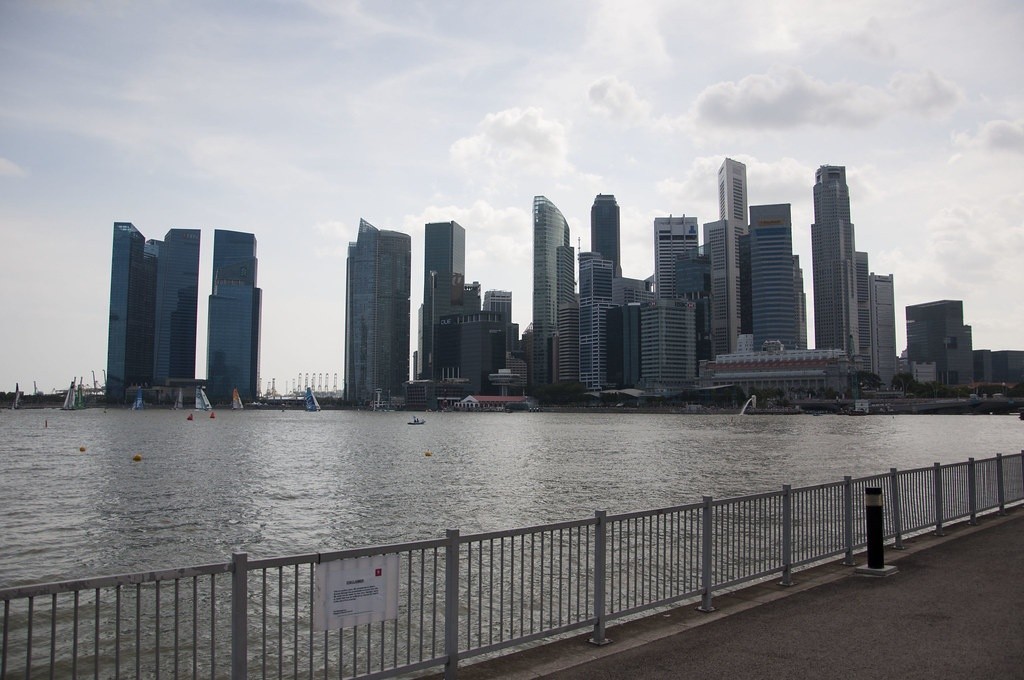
[407,415,426,425]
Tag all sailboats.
[170,387,183,410]
[194,384,214,411]
[304,387,322,411]
[128,385,143,411]
[56,375,86,411]
[231,387,246,411]
